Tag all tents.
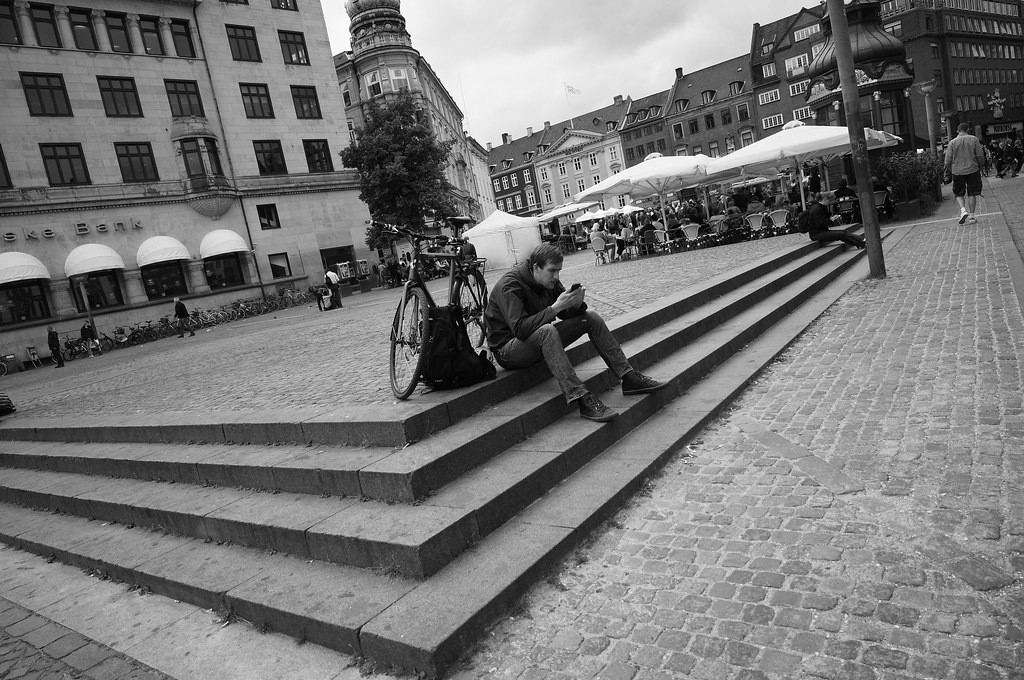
[575,210,596,223]
[593,209,606,220]
[460,209,542,269]
[604,207,623,216]
[618,205,644,215]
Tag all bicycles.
[365,216,488,400]
[51,287,328,364]
[381,252,450,290]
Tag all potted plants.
[338,278,353,298]
[357,275,370,293]
[877,150,923,221]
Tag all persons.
[485,247,669,421]
[315,268,343,311]
[81,320,99,357]
[941,139,1024,179]
[564,197,704,256]
[808,195,867,248]
[47,325,65,368]
[591,169,885,264]
[173,297,195,338]
[944,123,985,224]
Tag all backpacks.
[797,204,820,234]
[419,305,497,392]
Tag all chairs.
[590,190,895,266]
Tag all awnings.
[0,252,51,284]
[199,229,250,259]
[137,236,191,268]
[65,243,126,279]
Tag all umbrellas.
[630,154,760,202]
[704,120,903,213]
[744,131,904,192]
[535,202,599,233]
[573,152,742,241]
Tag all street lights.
[912,79,939,166]
[937,109,957,143]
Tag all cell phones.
[571,283,581,292]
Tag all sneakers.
[622,370,668,394]
[580,394,619,421]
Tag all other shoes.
[958,211,969,224]
[969,217,977,224]
